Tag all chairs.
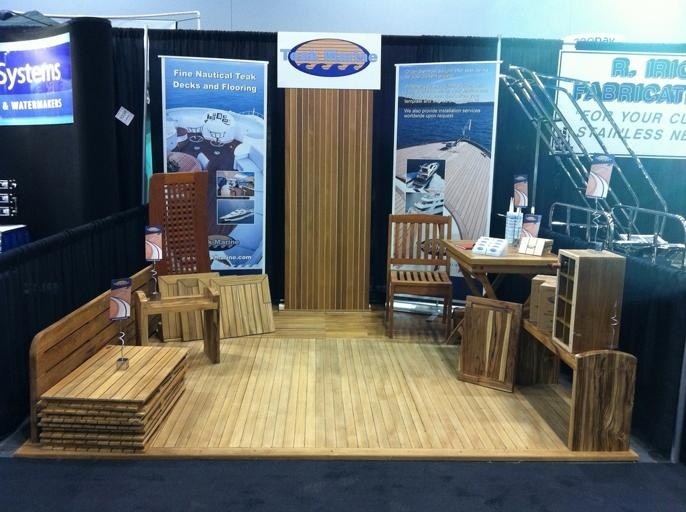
[385,212,453,337]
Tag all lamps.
[107,277,132,370]
[586,153,616,253]
[144,223,164,302]
[513,174,529,249]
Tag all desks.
[135,284,222,364]
[439,238,560,350]
[518,306,637,453]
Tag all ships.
[168,107,264,270]
[407,162,444,192]
[396,139,491,277]
[411,163,439,188]
